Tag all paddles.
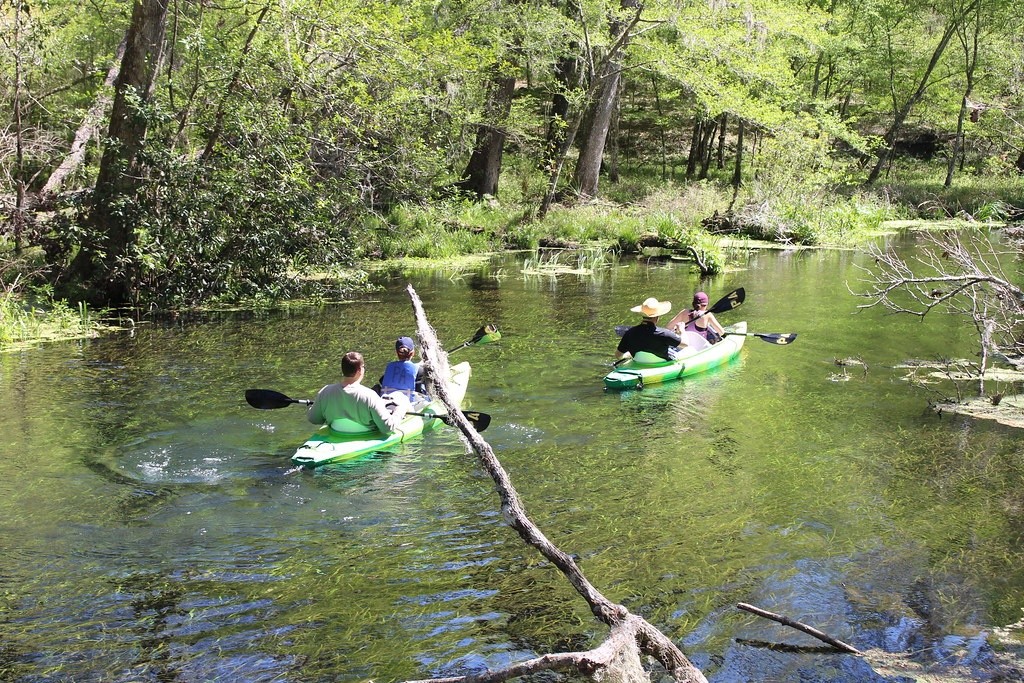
[615,287,745,366]
[615,317,798,346]
[372,324,502,398]
[244,389,491,434]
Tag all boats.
[290,361,473,469]
[603,320,748,402]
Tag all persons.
[665,292,725,343]
[308,352,408,436]
[380,337,425,403]
[615,298,689,362]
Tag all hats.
[395,337,415,351]
[630,298,671,318]
[693,292,709,305]
